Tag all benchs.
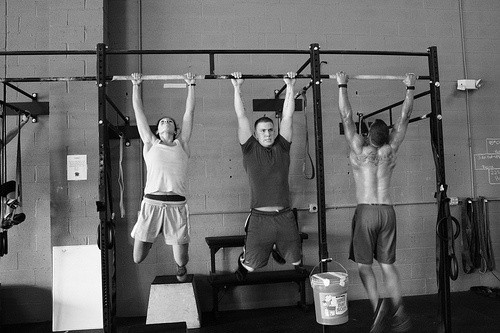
[205,232,310,321]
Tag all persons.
[129,72,197,281]
[335,71,420,333]
[230,72,302,282]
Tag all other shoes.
[391,305,404,319]
[369,299,385,332]
[235,252,248,284]
[272,249,286,264]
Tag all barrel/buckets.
[309,258,349,325]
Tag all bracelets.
[133,84,141,87]
[187,84,196,86]
[337,83,347,88]
[405,86,415,90]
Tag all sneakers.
[177,265,187,281]
[2,212,25,228]
